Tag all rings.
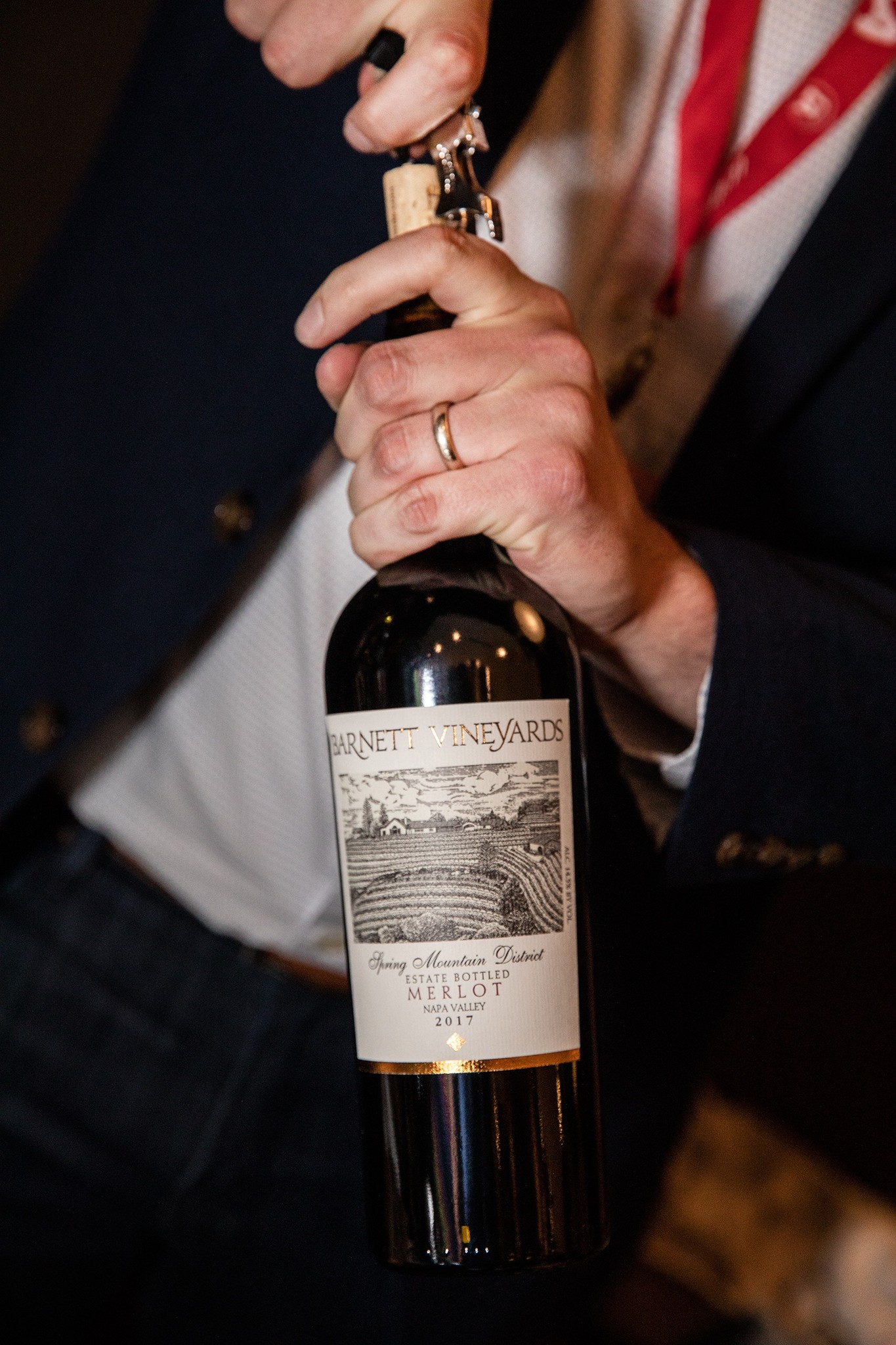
[430,400,470,472]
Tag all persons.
[0,2,896,1345]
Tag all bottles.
[323,289,608,1283]
